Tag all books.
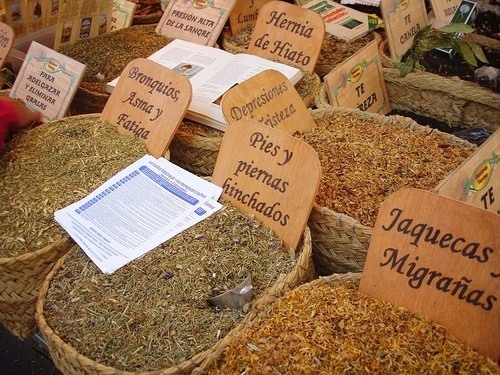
[109,38,307,133]
[301,0,384,45]
